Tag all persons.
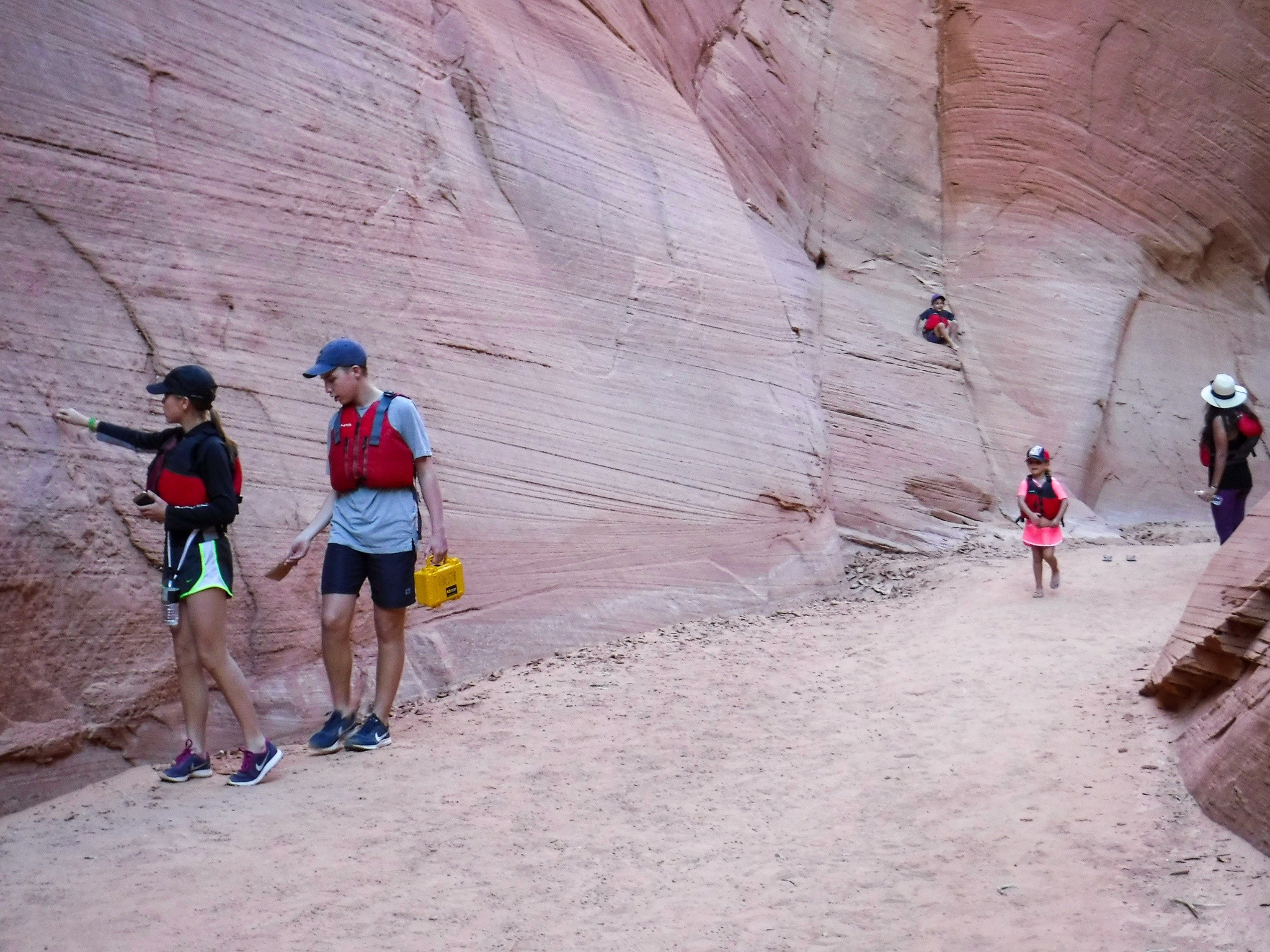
[915,293,960,352]
[284,337,448,754]
[1015,444,1070,598]
[51,366,283,786]
[1200,373,1261,546]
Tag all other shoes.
[1033,592,1044,598]
[1050,572,1061,588]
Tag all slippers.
[1102,555,1113,562]
[1126,555,1137,561]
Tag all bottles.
[160,570,180,625]
[1193,490,1223,506]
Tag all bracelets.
[87,415,98,433]
[1208,485,1218,489]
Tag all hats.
[1201,373,1248,408]
[1025,445,1050,463]
[303,336,366,379]
[932,293,946,304]
[147,366,219,400]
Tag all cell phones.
[132,491,155,506]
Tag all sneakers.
[227,737,284,786]
[307,709,358,753]
[159,738,213,782]
[345,713,392,750]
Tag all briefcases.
[414,554,464,609]
